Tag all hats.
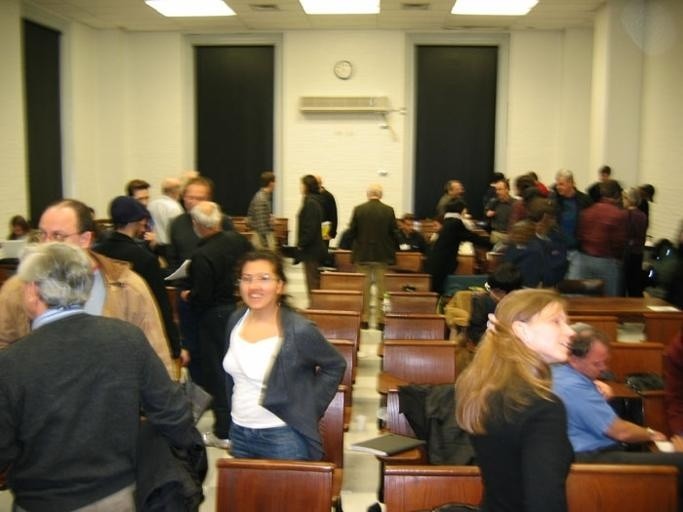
[108,194,149,226]
[638,183,656,205]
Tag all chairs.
[215,250,683,512]
[166,286,183,318]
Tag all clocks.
[333,60,354,80]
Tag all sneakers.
[375,322,386,332]
[360,321,370,330]
[200,431,232,451]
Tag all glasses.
[30,227,81,244]
[238,272,281,284]
